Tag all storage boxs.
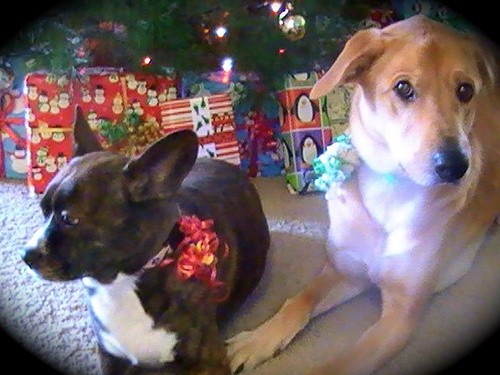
[1,65,355,195]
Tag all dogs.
[226,12,500,375]
[21,105,273,375]
